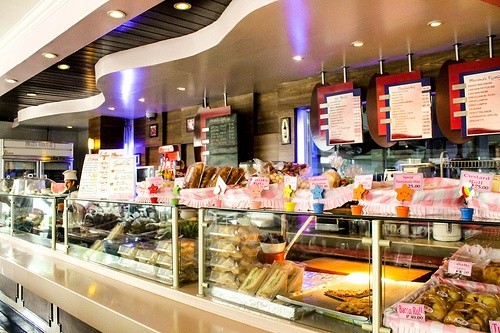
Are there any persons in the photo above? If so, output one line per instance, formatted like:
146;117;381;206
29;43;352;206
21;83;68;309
57;170;78;228
434;151;460;179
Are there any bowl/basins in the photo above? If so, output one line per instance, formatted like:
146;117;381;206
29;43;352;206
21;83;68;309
433;222;461;242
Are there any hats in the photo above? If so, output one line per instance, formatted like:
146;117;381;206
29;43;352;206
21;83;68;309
62;170;77;181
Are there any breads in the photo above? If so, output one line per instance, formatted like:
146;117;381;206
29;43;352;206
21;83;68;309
118;242;195;265
184;164;339;189
88;223;123;251
413;265;500;332
216;226;259;284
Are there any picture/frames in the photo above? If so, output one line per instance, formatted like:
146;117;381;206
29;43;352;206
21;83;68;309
186;117;194;132
280;117;291;145
149;124;158;137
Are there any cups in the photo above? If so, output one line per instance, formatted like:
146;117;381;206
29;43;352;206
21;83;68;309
257;251;285;264
260;241;286;254
385;225;432;238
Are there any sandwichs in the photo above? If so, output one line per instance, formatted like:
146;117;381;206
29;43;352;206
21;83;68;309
241;261;303;295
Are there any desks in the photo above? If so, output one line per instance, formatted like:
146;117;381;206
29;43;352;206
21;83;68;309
303;256;432;282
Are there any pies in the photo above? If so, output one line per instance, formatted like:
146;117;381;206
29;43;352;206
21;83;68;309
325;289;372;316
